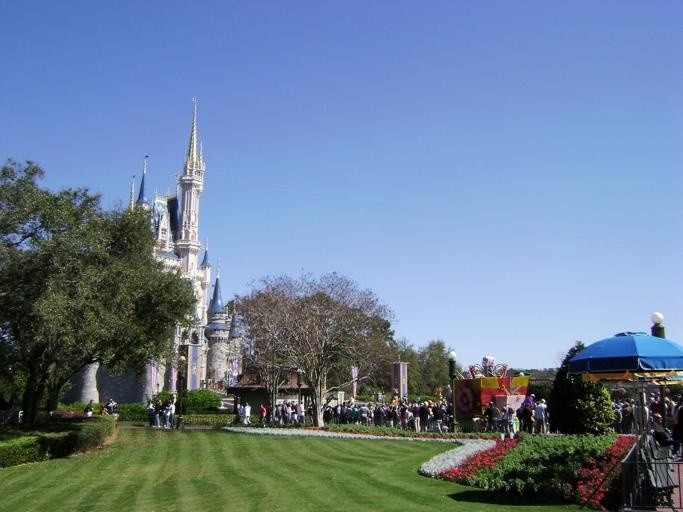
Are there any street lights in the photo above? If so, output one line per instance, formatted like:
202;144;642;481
175;355;186;429
225;368;232;387
447;351;457;434
649;311;667;429
208;366;212;379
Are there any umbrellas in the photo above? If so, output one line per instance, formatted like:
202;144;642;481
567;333;683;391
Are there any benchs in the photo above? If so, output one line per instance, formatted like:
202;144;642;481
637;424;679;512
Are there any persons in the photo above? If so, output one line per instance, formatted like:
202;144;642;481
517;394;547;434
233;397;251;424
349;395;355;407
611;391;683;455
325;400;449;434
483;401;514;433
259;404;272;423
146;394;182;429
84;400;94;416
100;399;117;415
